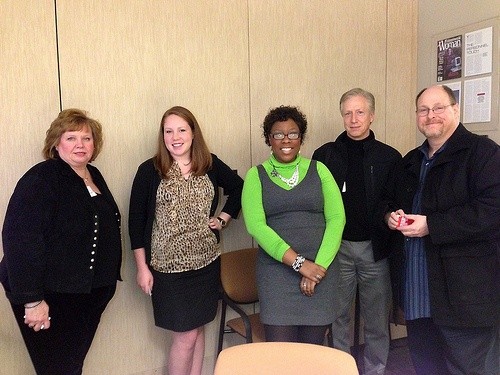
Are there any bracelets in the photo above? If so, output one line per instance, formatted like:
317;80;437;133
290;253;306;273
23;300;43;309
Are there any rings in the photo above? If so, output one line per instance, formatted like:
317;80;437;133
315;274;320;279
303;282;307;286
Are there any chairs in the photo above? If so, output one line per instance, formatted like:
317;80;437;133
214;339;361;375
215;248;266;358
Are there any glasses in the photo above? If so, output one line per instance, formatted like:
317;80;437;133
270;132;302;140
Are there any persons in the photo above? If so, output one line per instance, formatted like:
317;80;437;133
0;108;125;375
126;105;246;374
241;105;347;346
363;84;500;375
309;89;405;375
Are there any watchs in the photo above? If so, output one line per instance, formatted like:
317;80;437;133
217;217;226;230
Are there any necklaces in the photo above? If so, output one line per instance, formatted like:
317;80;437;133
267;155;301;187
70;166;88;181
